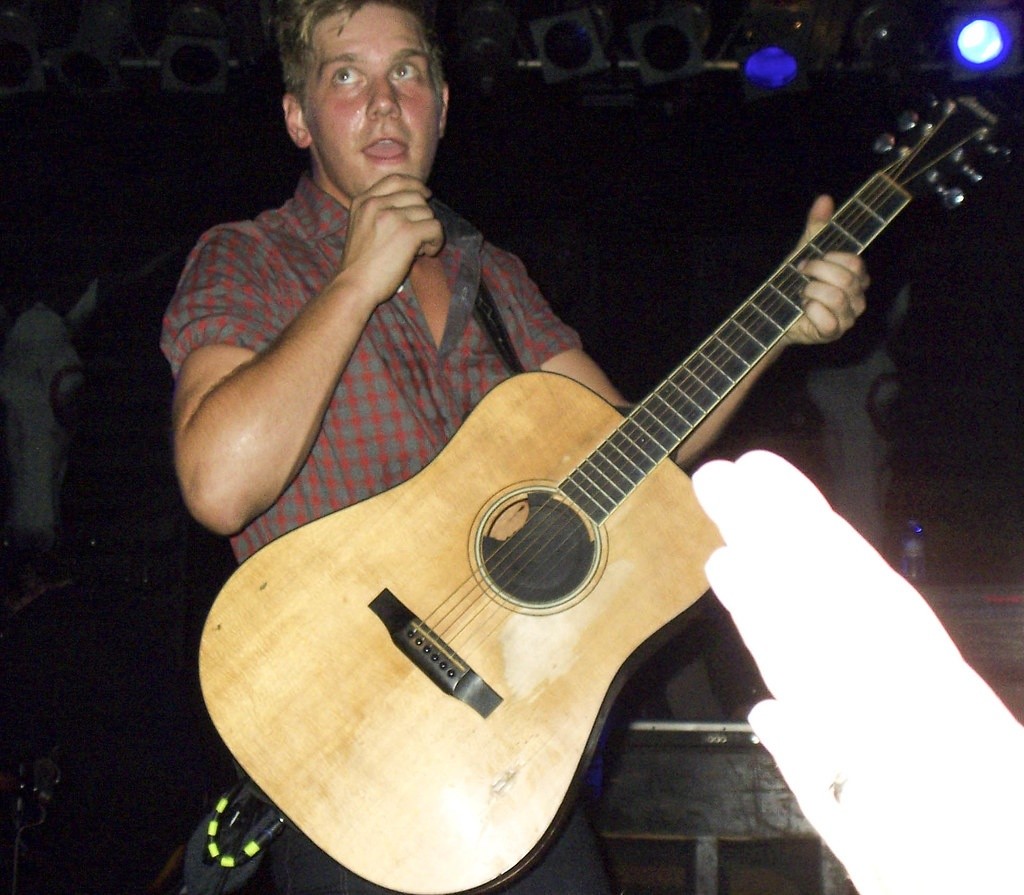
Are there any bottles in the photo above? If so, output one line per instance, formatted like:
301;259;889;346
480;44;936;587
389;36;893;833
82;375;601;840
900;538;937;613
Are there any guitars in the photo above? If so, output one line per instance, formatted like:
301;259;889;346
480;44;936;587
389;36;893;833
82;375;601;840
194;92;1016;893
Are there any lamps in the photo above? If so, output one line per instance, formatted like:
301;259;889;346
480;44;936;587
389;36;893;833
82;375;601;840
528;0;611;84
851;0;924;73
629;0;709;85
53;0;134;95
0;0;42;96
947;0;1024;79
161;0;229;94
733;0;844;102
459;0;518;70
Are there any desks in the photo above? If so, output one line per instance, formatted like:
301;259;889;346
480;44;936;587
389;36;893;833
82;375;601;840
592;586;1024;895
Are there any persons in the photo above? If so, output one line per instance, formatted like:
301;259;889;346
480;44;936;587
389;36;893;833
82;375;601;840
162;0;870;895
0;275;87;570
